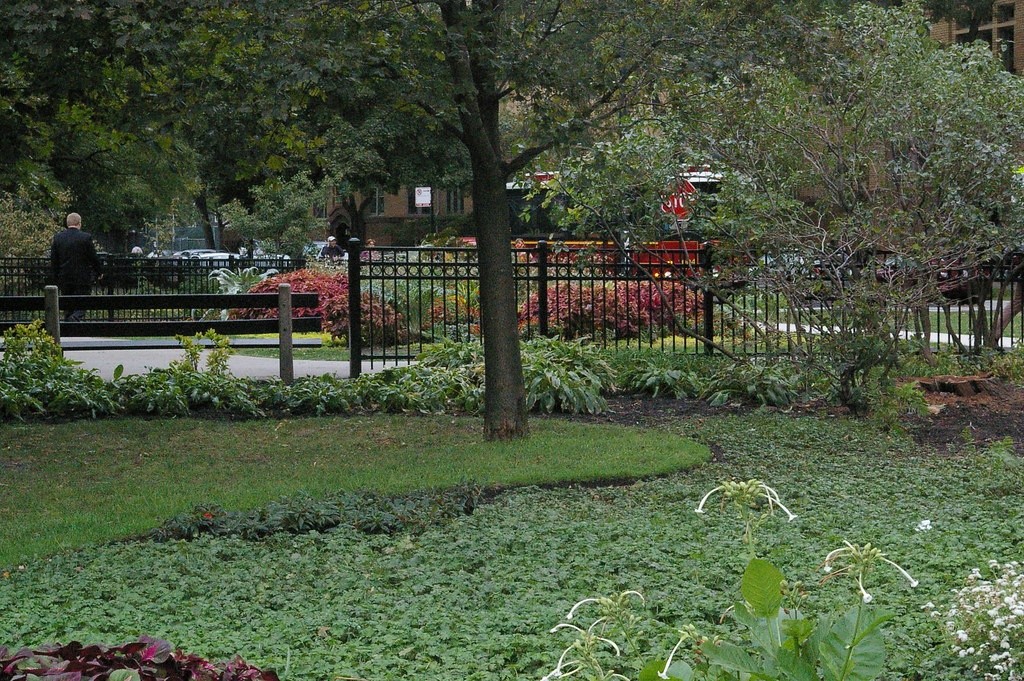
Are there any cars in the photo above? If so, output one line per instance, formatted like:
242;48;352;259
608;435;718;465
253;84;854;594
133;242;348;272
747;252;993;301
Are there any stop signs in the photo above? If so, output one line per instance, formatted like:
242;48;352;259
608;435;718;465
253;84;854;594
659;177;701;222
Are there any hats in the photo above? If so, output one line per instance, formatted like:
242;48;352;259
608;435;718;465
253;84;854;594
328;235;336;242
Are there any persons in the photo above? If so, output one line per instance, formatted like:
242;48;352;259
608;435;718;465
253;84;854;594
322;236;345;258
50;211;104;323
360;238;382;261
511;238;533;263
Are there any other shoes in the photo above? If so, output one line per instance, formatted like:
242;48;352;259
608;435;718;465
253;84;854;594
67;316;78;322
